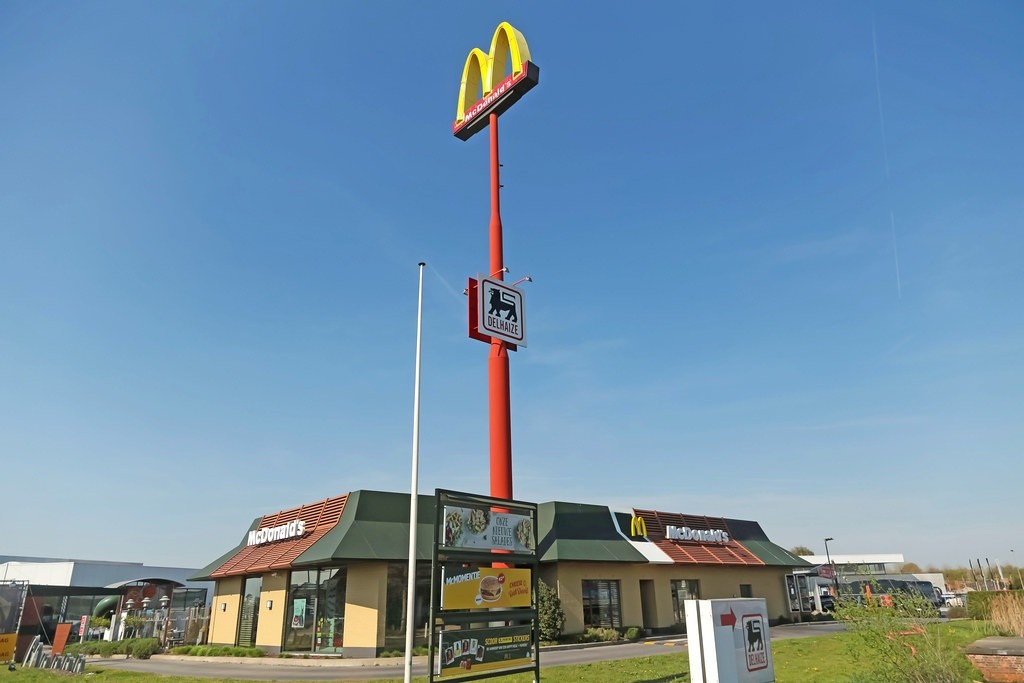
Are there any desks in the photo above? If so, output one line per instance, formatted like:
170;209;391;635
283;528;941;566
171;631;184;638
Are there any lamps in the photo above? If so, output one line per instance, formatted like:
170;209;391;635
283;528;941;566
159;595;170;606
125;599;135;609
221;603;226;612
141;597;151;609
193;598;200;607
267;600;272;609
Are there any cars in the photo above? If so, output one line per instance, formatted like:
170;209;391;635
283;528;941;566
945;596;964;608
808;594;840;612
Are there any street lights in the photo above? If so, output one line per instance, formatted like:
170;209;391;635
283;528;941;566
824;537;836;586
403;262;426;683
1010;548;1024;590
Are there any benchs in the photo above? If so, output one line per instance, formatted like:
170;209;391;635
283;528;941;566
168;639;184;647
